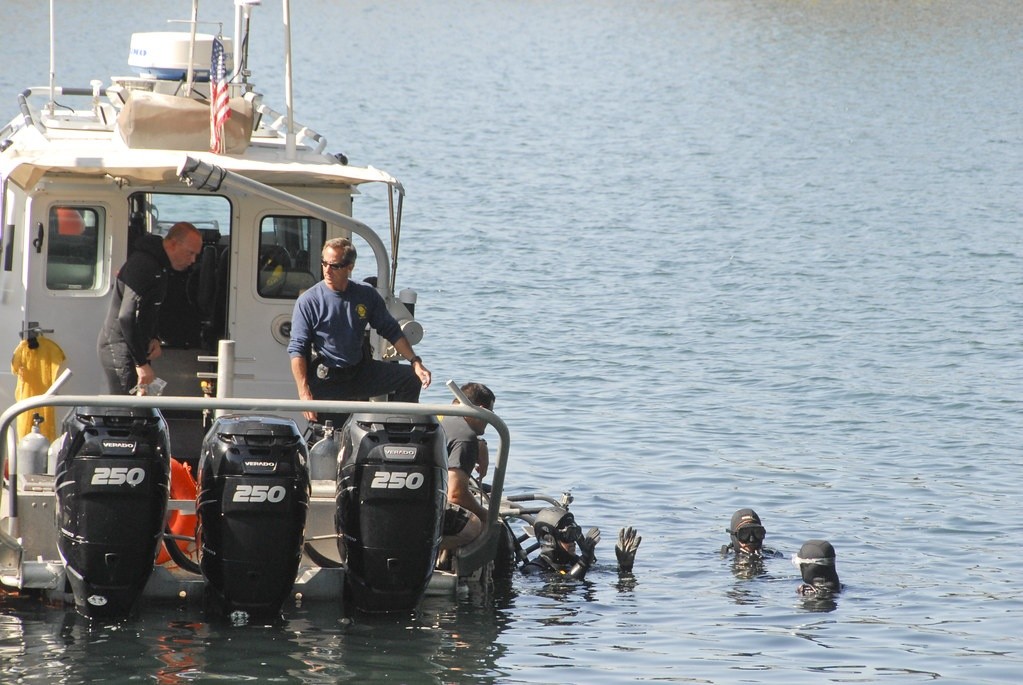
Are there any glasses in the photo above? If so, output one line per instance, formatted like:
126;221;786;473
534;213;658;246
558;523;582;541
321;259;350;270
792;553;811;568
735;524;766;542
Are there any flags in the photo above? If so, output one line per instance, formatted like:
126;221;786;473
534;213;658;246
210;35;232;154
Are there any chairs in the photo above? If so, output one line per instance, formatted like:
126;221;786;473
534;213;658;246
129;225;311;351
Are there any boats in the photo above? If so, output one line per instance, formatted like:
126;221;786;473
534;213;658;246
1;0;573;623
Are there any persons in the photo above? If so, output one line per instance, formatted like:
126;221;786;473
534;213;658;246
720;508;783;557
791;539;845;594
96;221;203;395
286;237;432;451
520;506;642;578
441;382;514;576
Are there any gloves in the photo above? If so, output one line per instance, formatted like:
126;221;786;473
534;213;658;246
582;528;601;566
615;526;641;571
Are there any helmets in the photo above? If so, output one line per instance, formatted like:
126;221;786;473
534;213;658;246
56;208;87;236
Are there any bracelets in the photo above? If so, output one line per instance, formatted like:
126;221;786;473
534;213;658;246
410;356;422;364
